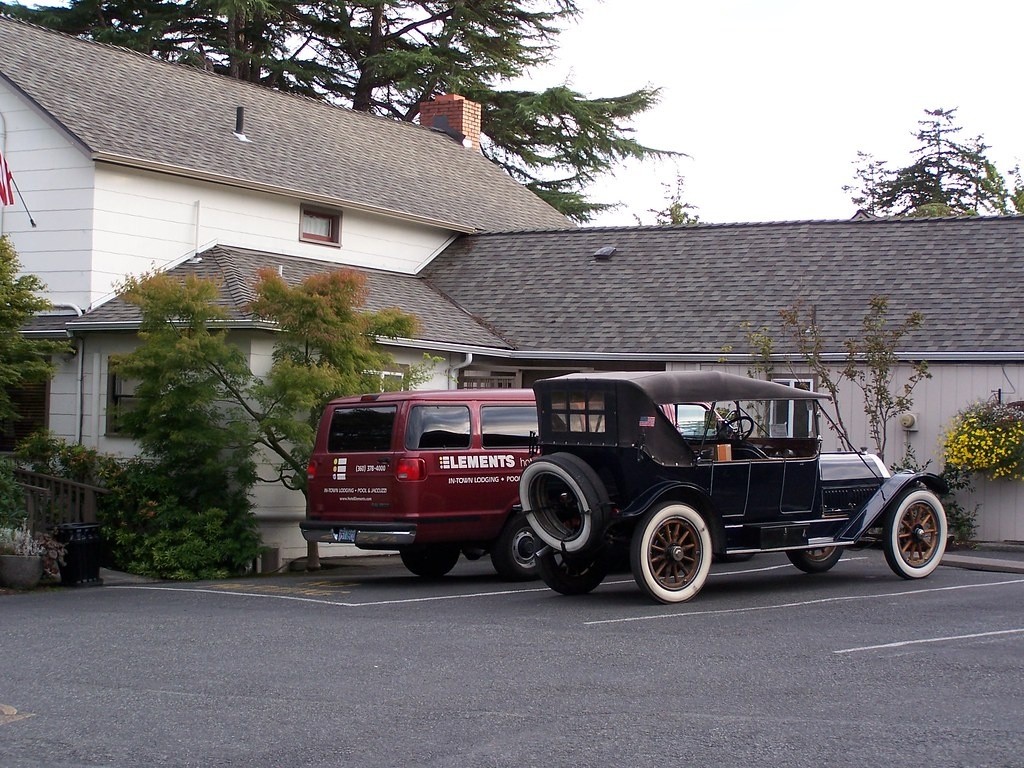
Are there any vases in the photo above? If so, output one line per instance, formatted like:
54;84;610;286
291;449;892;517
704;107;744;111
0;554;42;590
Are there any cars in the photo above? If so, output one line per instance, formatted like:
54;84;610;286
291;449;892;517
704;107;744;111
514;371;949;605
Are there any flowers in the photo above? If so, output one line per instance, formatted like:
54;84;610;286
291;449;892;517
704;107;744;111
0;517;46;555
934;397;1024;483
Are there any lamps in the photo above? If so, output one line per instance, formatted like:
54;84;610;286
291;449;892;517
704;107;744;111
901;411;920;432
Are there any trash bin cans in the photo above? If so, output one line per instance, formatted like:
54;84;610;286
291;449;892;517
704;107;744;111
47;522;104;587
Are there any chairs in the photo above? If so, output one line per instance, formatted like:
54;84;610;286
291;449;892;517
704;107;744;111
727;439;767;458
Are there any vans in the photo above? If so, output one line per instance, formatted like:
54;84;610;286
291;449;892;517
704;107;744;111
298;384;740;583
